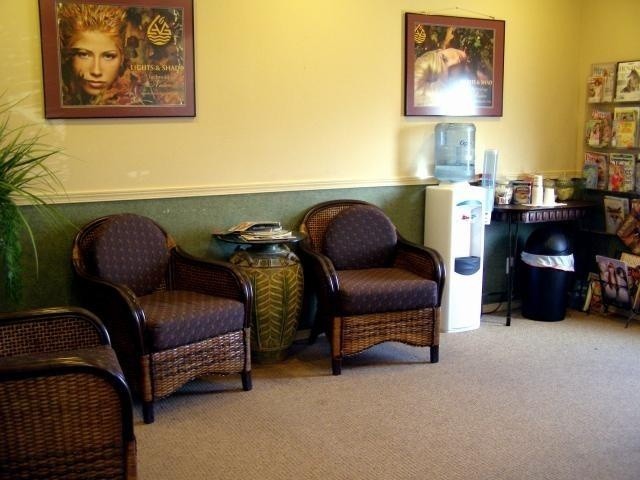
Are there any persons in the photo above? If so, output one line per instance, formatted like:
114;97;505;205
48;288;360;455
56;3;140;106
414;49;494;112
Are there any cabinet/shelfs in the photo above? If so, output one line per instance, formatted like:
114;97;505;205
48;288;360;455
583;58;640;329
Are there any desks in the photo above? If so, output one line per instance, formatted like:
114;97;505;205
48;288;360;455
210;223;311;369
479;192;591;324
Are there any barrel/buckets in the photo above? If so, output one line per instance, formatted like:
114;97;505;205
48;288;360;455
434;123;476;183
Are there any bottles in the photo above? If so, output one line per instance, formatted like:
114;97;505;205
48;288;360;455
568;178;586;201
495;180;514;205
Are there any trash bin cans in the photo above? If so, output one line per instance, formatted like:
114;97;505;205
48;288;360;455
521;228;571;322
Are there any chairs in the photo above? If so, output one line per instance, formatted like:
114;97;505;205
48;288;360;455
2;304;141;479
298;196;447;377
67;211;257;424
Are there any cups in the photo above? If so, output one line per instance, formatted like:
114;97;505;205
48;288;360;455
531;174;555;206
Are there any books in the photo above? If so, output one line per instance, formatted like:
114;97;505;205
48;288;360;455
581;61;639;317
214;220;298;240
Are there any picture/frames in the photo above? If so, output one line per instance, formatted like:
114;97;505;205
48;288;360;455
37;0;199;121
405;10;507;118
